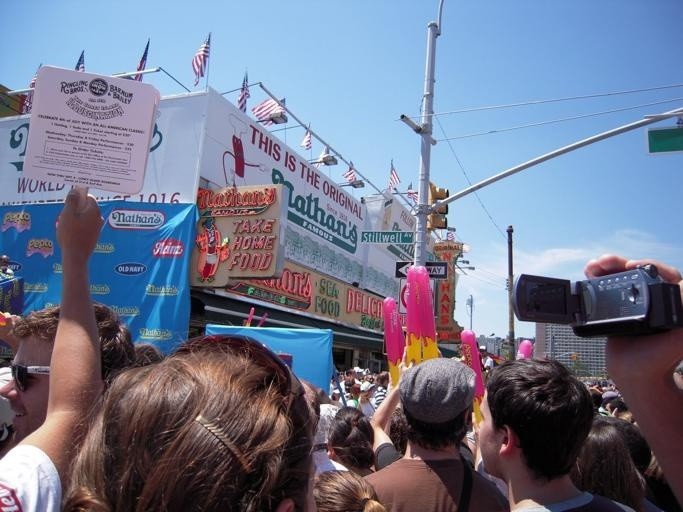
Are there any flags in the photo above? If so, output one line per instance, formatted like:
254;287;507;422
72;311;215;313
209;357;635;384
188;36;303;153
21;63;42;116
341;161;356;183
251;96;284;128
74;50;84;72
412;192;418;203
387;167;401;188
237;74;249;113
191;33;210;85
132;40;150;82
298;126;311;150
407;184;411;198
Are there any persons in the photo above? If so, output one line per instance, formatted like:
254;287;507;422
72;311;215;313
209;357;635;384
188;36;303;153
582;254;682;511
0;186;109;512
0;298;681;512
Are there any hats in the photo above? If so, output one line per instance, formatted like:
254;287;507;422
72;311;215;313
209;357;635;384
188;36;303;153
354;366;375;392
601;391;619;400
399;358;475;424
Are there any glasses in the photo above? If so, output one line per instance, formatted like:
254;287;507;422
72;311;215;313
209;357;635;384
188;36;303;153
10;361;51;391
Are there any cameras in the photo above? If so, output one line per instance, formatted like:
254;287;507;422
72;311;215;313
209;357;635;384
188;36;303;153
510;263;682;338
